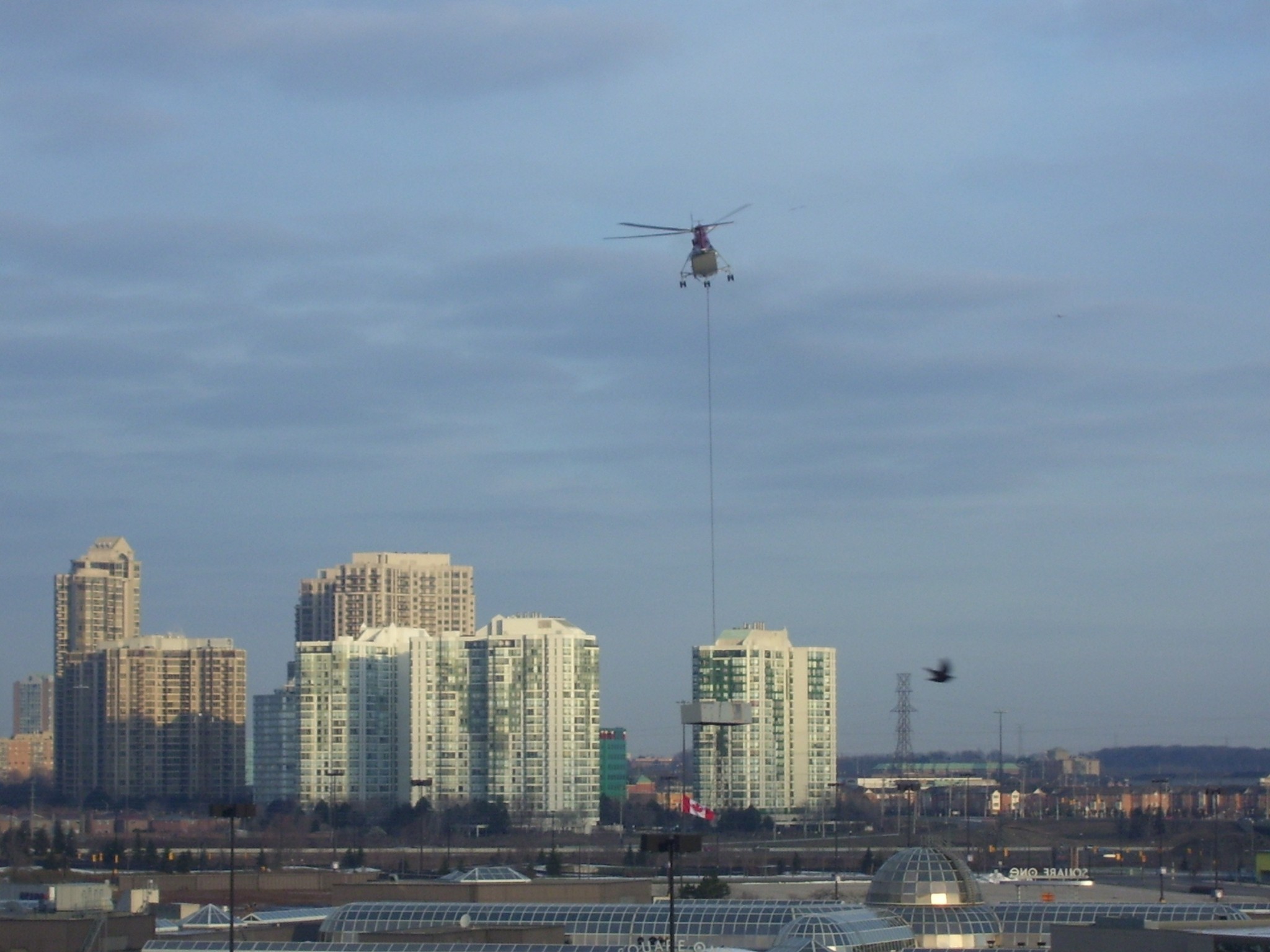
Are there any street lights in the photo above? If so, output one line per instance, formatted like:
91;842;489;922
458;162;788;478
1150;778;1170;904
219;804;240;952
989;709;1008;839
826;782;847;900
321;768;346;862
959;771;978;860
895;781;922;848
1205;788;1222;895
406;776;432;878
637;828;704;952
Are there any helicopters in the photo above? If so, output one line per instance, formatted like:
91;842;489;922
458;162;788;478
603;193;757;287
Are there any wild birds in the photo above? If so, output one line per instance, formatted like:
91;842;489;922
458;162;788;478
923;658;956;682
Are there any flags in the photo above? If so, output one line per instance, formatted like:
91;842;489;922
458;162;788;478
681;794;715;821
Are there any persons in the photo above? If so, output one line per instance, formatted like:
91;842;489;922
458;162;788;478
637;935;669;946
